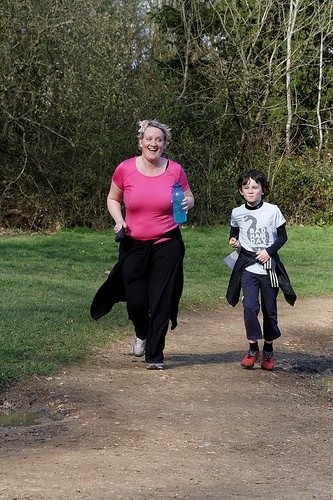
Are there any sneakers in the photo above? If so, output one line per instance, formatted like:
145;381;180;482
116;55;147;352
146;362;164;370
262;352;275;370
133;337;146;357
240;349;261;368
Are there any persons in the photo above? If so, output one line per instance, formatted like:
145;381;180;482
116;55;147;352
89;121;195;370
225;169;298;371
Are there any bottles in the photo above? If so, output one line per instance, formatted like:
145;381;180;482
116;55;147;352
172;182;188;223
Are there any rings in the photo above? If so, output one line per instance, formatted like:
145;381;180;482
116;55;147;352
115;227;117;228
